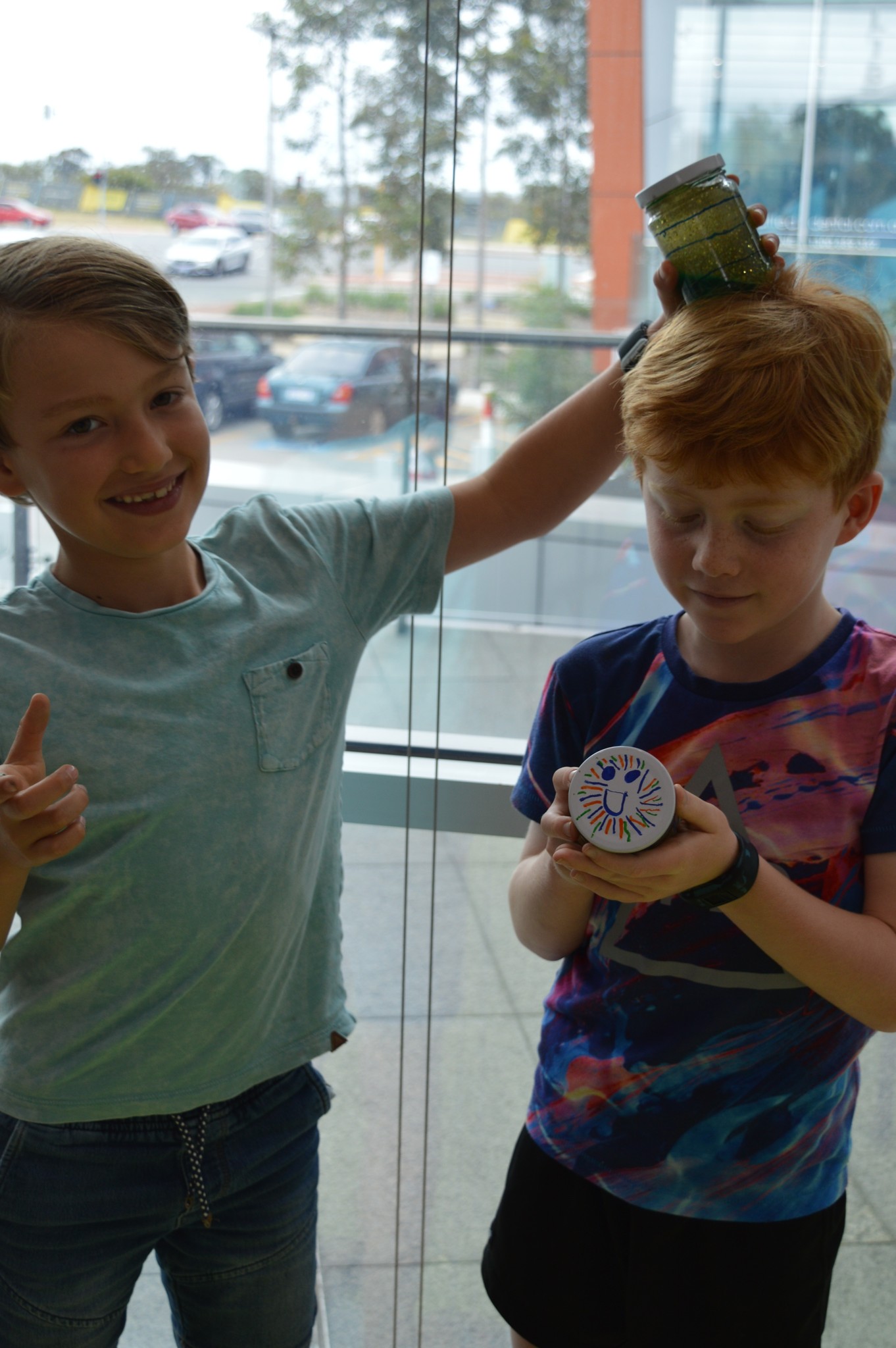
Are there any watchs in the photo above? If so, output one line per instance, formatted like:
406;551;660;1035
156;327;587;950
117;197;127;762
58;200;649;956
694;828;759;915
617;319;656;375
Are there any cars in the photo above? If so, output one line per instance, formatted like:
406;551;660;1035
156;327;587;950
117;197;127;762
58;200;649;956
0;194;54;226
249;336;460;443
164;202;316;279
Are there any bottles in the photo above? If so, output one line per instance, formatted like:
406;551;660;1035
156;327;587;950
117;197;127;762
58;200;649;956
636;152;773;309
569;745;680;858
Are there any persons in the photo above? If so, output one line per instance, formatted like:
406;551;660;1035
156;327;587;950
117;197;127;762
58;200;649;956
480;264;896;1348
0;174;790;1348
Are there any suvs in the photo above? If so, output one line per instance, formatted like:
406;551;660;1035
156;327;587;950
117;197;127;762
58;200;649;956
183;327;282;431
